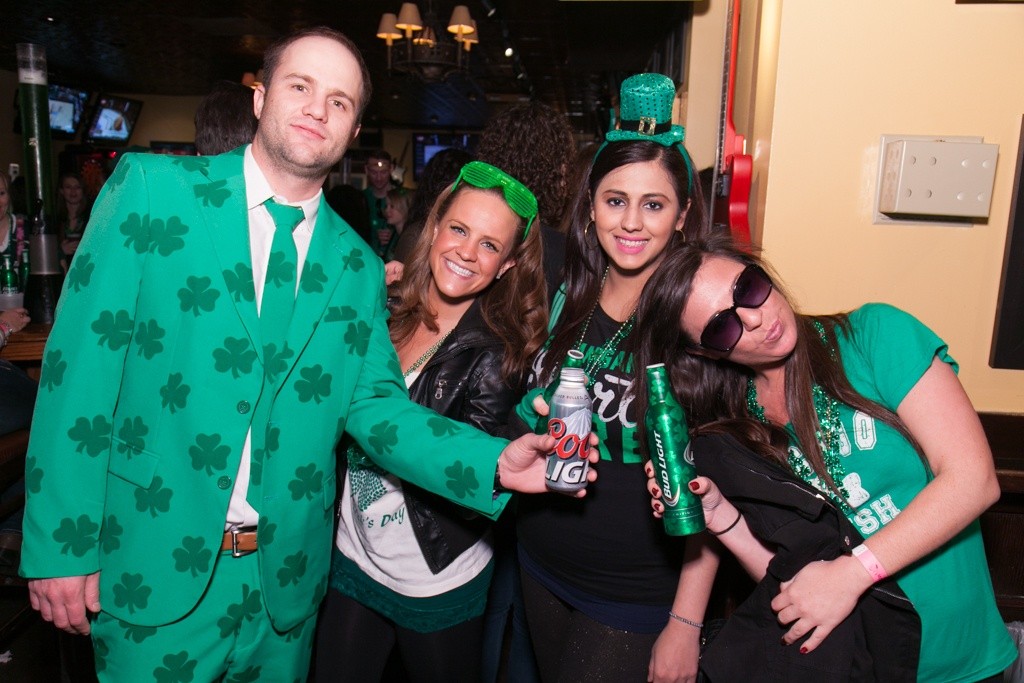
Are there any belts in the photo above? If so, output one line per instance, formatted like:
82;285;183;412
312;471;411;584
220;525;259;558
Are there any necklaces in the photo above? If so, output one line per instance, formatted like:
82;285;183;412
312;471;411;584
558;261;657;393
402;326;456;380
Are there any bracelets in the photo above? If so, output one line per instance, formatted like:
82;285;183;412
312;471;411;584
714;512;742;538
0;319;12;346
669;612;704;629
852;544;887;583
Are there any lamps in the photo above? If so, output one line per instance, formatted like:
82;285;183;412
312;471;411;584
479;1;539;96
377;2;479;83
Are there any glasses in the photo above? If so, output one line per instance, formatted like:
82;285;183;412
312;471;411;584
697;264;773;354
452;161;538;242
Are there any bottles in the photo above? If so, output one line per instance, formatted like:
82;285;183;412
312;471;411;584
19;248;29;292
644;362;706;537
1;253;18;295
536;349;584;436
380;222;388;254
544;368;592;492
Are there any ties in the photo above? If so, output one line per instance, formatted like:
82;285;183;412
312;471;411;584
246;198;306;515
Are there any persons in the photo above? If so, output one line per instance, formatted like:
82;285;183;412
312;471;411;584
55;170;92;276
638;239;1019;683
363;150;409;258
512;128;721;683
385;186;419;262
475;102;583;316
17;26;600;683
194;89;258;158
0;171;34;293
327;184;380;254
0;308;31;352
321;161;550;682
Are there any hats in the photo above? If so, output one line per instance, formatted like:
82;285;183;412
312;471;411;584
605;73;684;147
